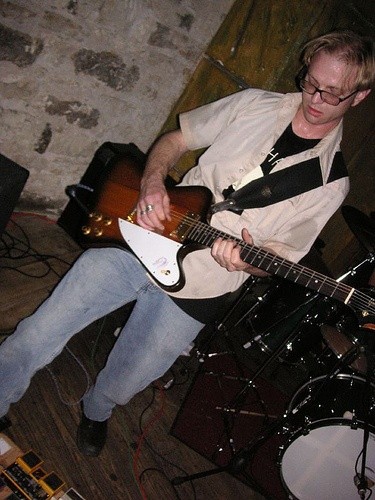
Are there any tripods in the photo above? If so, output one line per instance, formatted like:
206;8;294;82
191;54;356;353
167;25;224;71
171;250;375;500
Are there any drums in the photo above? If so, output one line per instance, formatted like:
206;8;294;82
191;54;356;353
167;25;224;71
277;374;374;500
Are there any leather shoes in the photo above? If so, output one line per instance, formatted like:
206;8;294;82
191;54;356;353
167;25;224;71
78;399;108;459
0;414;12;432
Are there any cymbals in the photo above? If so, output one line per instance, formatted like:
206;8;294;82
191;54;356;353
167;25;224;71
319;323;371;373
341;204;374;257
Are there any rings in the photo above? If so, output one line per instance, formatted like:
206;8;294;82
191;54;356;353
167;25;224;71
146;205;154;212
141;211;146;216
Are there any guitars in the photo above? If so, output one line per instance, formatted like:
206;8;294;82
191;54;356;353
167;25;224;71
56;140;375;318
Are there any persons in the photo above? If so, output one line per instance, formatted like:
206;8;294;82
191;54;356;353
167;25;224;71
0;32;371;458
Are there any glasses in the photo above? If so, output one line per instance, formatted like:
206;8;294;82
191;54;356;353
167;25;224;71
297;64;359;106
